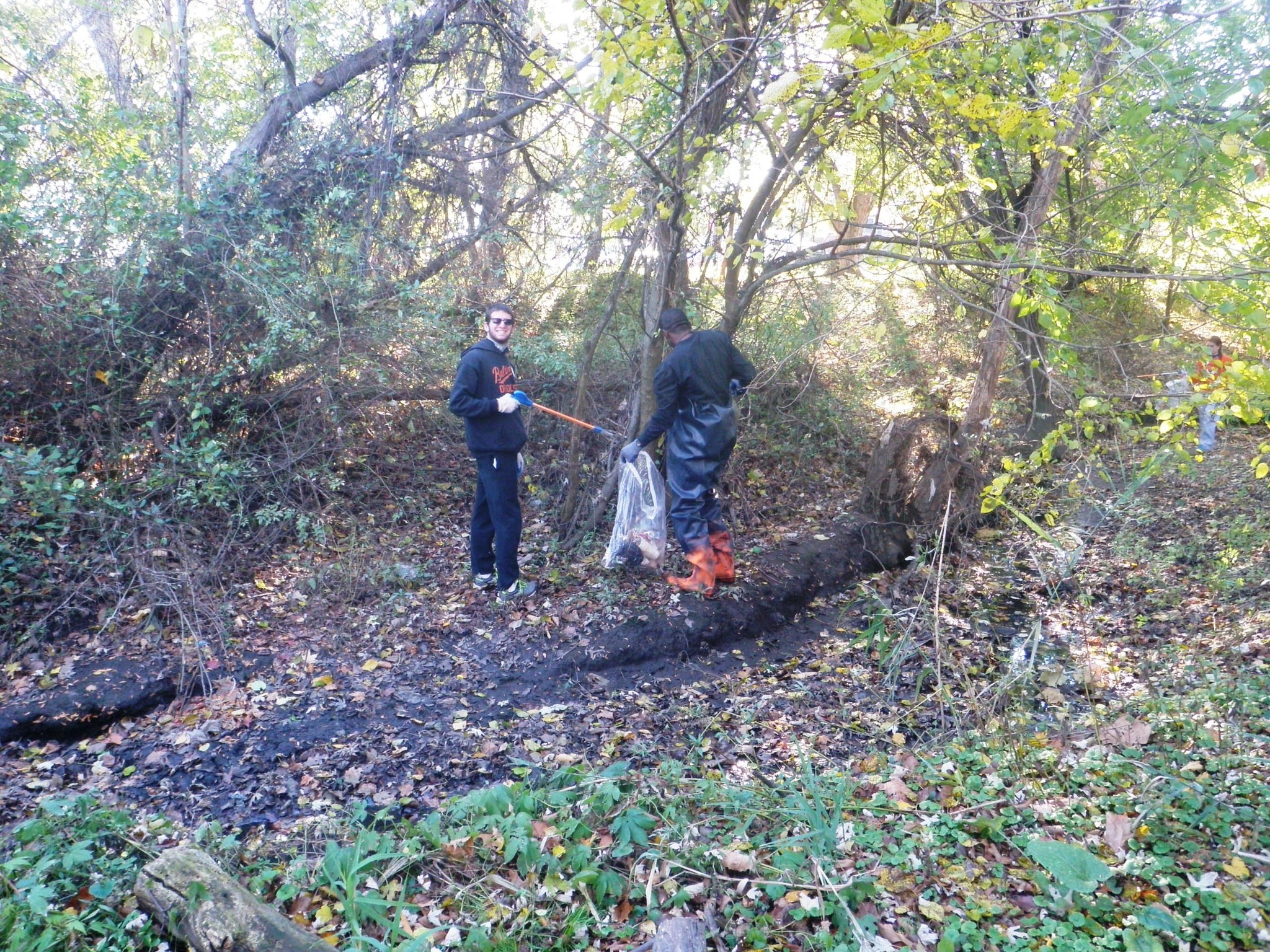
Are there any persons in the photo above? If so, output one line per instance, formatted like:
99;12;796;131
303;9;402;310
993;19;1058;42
1182;332;1234;453
617;303;757;603
447;300;543;603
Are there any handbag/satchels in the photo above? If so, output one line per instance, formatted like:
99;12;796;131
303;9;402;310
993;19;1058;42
601;450;667;576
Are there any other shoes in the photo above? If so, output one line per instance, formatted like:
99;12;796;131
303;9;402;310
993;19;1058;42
1198;444;1209;452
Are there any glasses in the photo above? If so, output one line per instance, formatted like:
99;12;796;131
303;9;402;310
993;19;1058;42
488;317;515;325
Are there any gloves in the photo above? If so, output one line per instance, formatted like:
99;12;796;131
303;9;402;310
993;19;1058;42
496;393;521;414
516;452;524;477
620;438;643;464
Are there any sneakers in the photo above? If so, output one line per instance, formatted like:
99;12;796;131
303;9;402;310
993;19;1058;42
472;568;498;590
495;578;538;606
